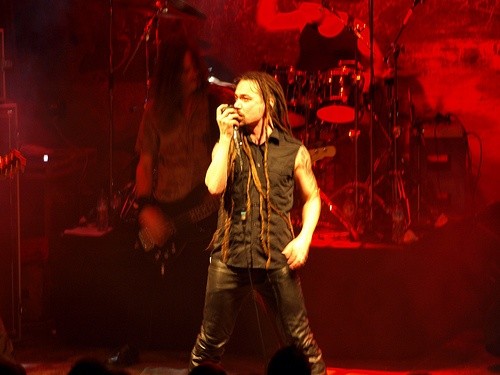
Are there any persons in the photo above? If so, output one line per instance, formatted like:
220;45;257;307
256;0;385;94
191;72;327;375
108;33;233;366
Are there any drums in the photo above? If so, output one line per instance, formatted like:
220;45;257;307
316;68;359;122
267;68;310;127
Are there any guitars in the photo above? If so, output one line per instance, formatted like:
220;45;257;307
134;195;222;253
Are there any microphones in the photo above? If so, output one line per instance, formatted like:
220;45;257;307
221;107;238;138
413;0;420;8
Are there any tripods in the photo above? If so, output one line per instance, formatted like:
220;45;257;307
349;0;424;243
